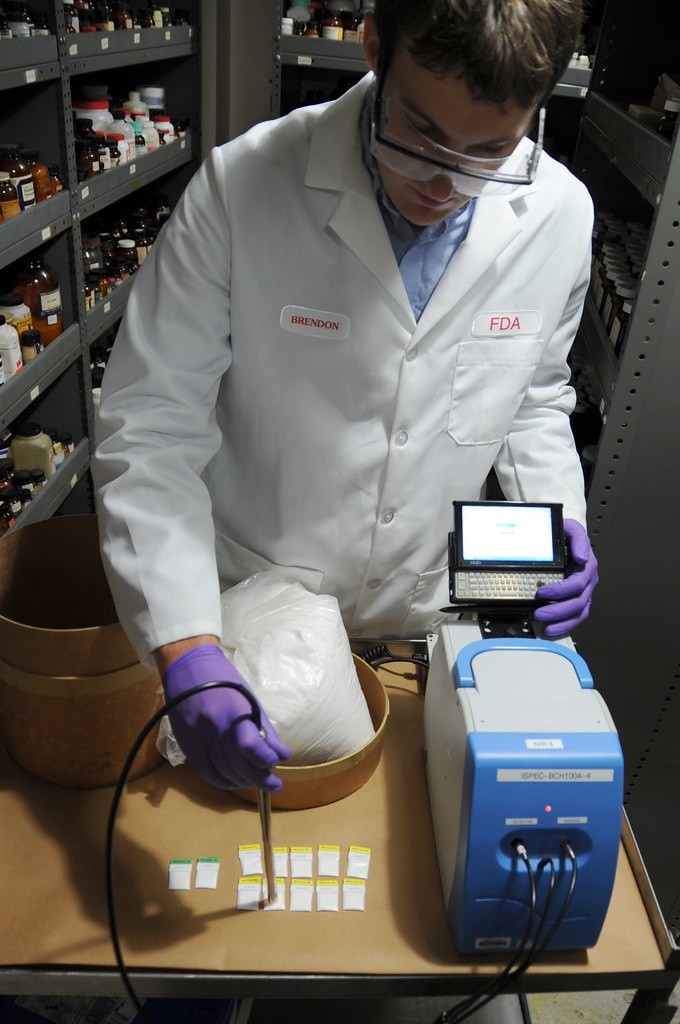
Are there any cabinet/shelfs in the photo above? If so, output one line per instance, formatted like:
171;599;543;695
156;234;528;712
0;0;232;539
570;0;680;946
269;0;593;124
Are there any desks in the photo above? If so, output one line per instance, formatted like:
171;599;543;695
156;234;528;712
0;637;680;1024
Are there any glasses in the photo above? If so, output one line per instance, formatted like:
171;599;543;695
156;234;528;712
369;70;545;196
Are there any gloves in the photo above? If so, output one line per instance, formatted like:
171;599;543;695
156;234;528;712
535;518;599;634
162;645;290;793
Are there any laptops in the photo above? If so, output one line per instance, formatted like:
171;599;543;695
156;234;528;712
451;500;566;604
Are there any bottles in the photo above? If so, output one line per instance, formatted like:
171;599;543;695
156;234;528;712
280;0;375;44
74;83;187;185
0;142;64;226
90;338;114;405
656;96;680;141
61;0;175;35
0;0;51;38
568;346;598;412
0;258;63;390
0;422;77;538
579;444;597;463
575;206;655;353
83;197;174;312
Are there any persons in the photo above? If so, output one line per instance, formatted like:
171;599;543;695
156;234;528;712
89;0;602;792
